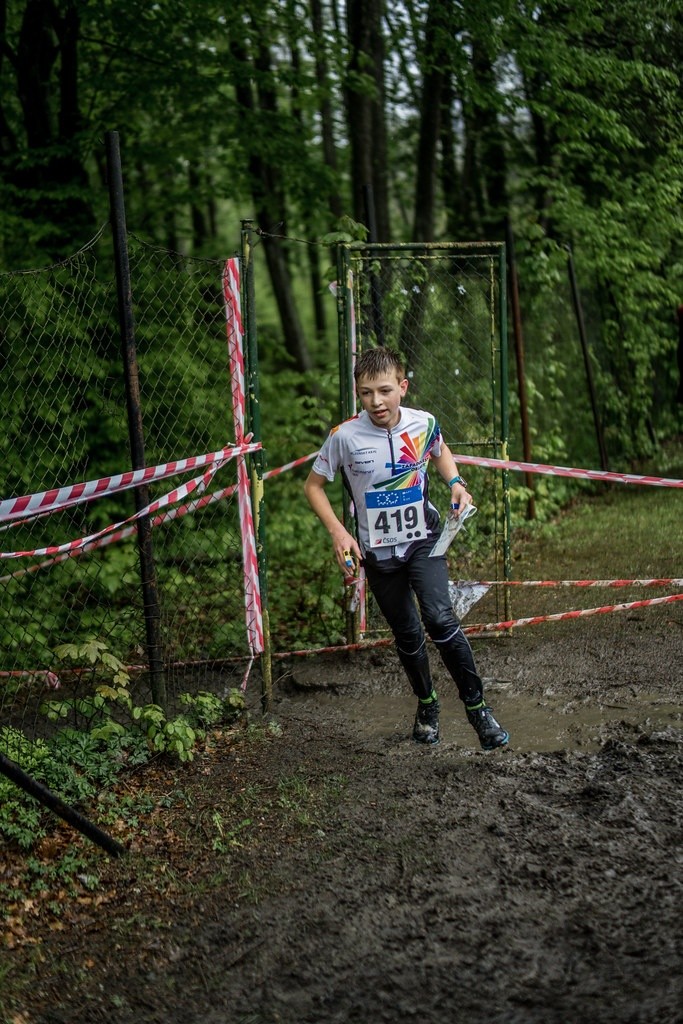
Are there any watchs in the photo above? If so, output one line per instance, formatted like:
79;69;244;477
448;475;467;489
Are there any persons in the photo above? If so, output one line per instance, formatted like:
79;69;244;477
303;348;513;751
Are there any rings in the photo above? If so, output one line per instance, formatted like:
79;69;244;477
342;549;354;567
449;503;459;510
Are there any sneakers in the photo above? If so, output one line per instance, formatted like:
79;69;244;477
465;698;510;750
412;689;440;744
